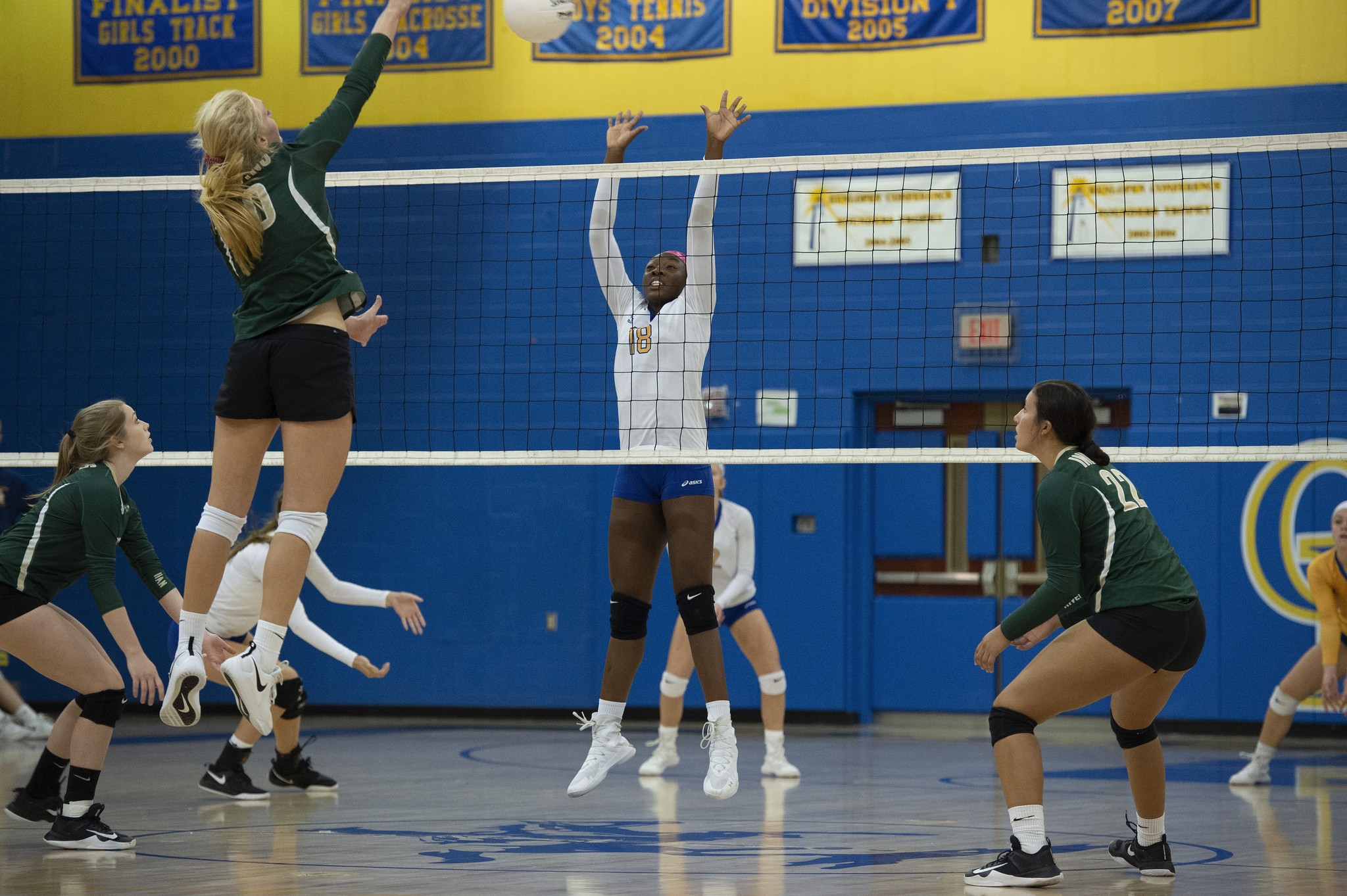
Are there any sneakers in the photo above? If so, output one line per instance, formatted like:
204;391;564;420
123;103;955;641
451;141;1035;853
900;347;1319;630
267;735;338;793
638;732;681;776
43;802;136;850
566;711;636;798
1108;810;1176;876
1228;751;1272;785
220;639;290;736
3;776;64;824
759;742;800;778
159;636;207;727
963;835;1063;887
700;712;739;800
197;763;271;801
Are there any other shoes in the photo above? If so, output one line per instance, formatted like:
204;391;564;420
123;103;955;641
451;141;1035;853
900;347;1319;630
1;710;55;741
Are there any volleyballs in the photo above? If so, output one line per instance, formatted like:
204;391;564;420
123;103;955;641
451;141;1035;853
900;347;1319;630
503;0;576;44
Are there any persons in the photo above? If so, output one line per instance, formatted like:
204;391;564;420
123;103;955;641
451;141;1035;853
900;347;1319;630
961;381;1210;886
158;0;424;803
0;397;236;851
1227;501;1347;788
555;88;802;797
